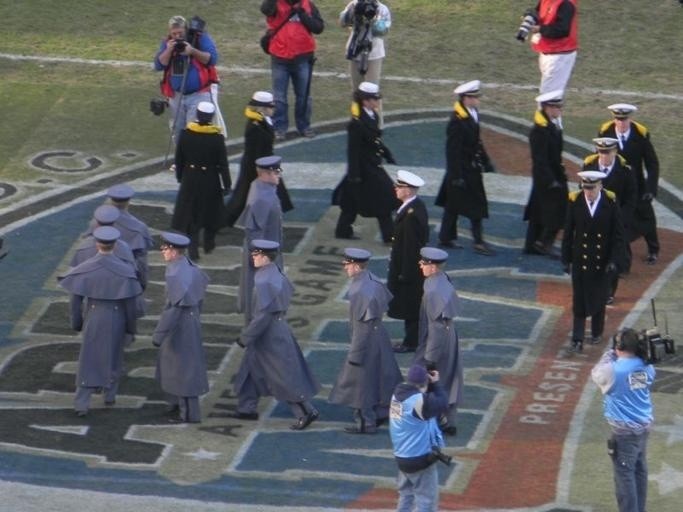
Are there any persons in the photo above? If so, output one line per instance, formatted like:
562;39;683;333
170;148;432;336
520;89;661;356
380;169;428;353
151;232;211;423
519;0;579;132
232;155;285;327
388;364;449;512
260;0;324;142
327;248;405;434
57;184;154;419
339;0;393;134
416;247;463;435
232;239;319;430
433;79;498;256
151;16;292;263
590;327;657;511
331;81;403;243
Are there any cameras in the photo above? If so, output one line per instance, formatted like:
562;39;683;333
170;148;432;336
173;37;186;52
362;1;375;18
515;11;538;41
425;363;436;374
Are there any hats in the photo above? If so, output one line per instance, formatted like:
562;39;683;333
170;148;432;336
408;365;428;382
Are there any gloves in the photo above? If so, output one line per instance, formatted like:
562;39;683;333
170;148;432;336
641;192;653;202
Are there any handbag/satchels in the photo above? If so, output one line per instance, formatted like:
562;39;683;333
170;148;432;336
261;29;274;54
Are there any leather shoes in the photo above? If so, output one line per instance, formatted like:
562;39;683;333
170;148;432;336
569;341;583;352
394;345;413;353
645;251;659;264
606;297;615;305
590;335;602;344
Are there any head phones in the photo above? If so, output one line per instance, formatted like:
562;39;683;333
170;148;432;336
616;329;625;352
